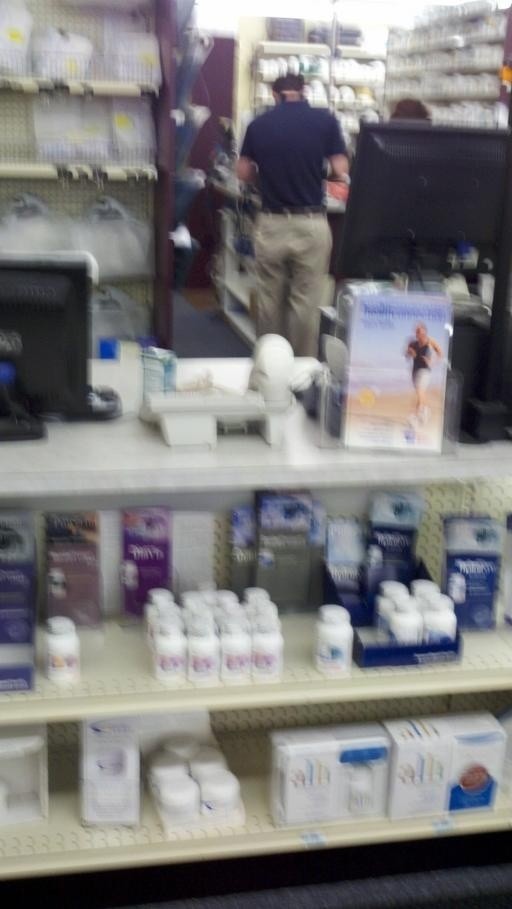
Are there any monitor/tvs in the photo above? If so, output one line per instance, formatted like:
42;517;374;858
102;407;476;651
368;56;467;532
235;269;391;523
0;255;94;440
333;117;511;287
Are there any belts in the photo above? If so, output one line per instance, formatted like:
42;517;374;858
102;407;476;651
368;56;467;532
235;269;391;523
262;204;324;215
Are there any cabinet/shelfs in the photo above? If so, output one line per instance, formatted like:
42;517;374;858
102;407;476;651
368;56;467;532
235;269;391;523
1;1;512;909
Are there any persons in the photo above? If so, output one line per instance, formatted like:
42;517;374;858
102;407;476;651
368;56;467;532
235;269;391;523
237;70;352;365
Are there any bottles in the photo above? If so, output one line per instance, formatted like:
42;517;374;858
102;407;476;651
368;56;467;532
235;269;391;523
43;614;80;683
144;587;284;686
372;574;460;645
314;603;355;674
